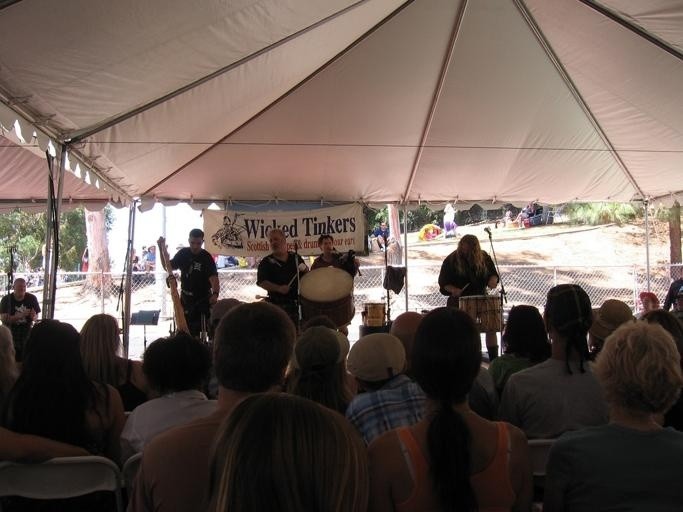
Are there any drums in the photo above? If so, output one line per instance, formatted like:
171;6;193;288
361;302;386;326
299;267;355;329
459;295;504;333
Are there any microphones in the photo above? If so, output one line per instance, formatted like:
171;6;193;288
383;234;388;247
484;227;491;235
294;240;298;255
128;240;131;250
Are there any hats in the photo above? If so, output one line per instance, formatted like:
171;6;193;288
544;284;595;343
211;297;247;327
346;332;407;383
587;298;638;341
392;312;423;343
639;292;661;304
294;325;351;371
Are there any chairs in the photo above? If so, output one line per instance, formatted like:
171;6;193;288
0;309;683;512
